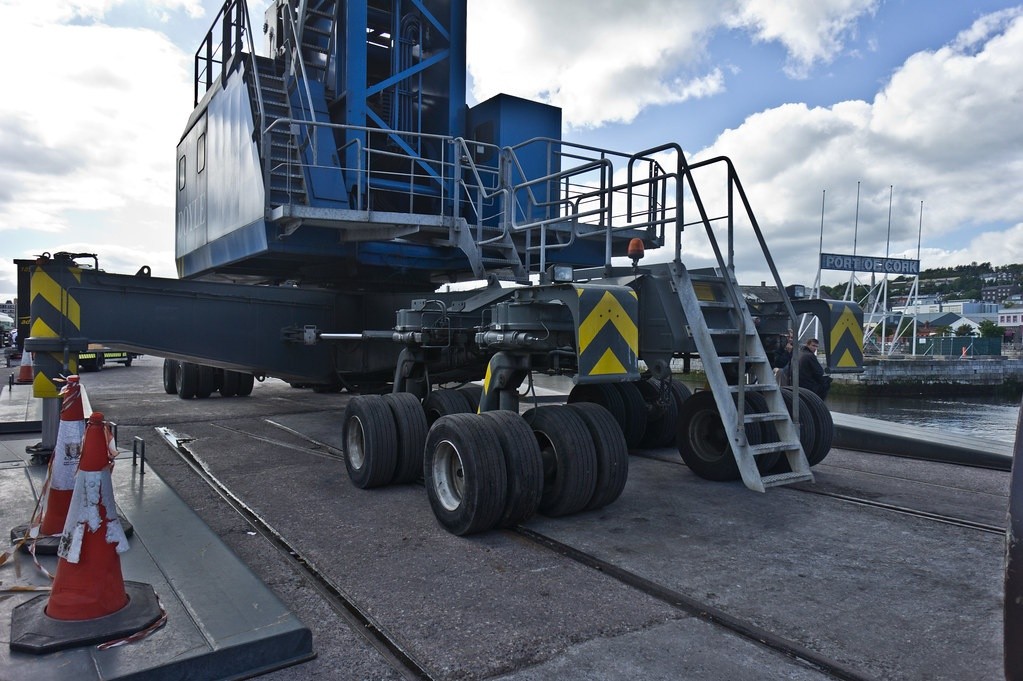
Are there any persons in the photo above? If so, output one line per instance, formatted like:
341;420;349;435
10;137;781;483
772;329;795;370
790;338;832;402
2;330;12;348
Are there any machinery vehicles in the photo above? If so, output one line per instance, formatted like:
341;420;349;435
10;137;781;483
24;1;865;537
10;374;133;556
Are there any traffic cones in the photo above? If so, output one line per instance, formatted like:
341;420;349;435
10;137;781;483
10;349;33;386
9;412;167;657
